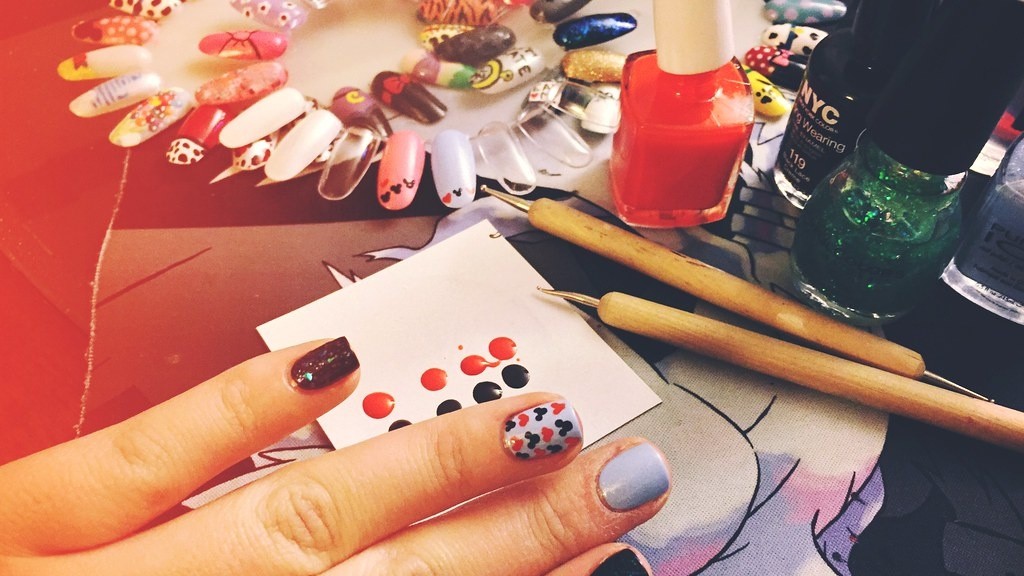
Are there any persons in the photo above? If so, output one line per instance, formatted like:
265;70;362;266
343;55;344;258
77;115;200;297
0;335;673;576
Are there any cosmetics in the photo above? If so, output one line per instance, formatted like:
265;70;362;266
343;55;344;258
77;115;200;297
608;0;756;230
773;0;1024;327
